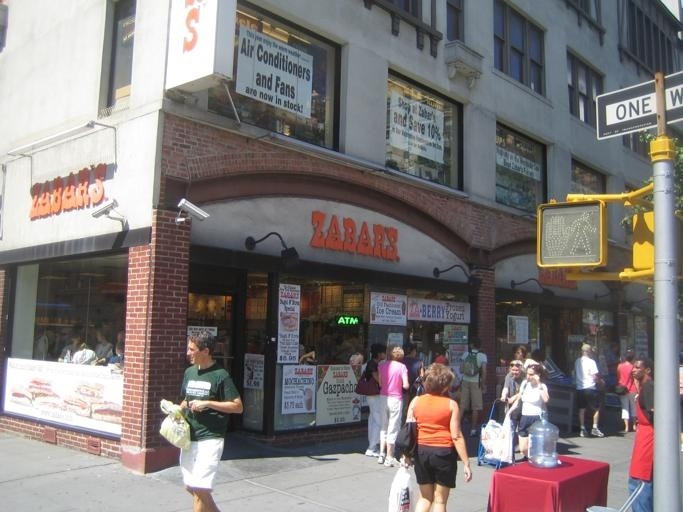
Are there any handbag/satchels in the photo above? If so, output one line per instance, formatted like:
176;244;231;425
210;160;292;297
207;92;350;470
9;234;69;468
614;385;628;395
511;399;523;421
395;421;415;459
354;372;381;396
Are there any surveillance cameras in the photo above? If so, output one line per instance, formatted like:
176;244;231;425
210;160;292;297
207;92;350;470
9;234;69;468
178;198;210;221
92;198;118;219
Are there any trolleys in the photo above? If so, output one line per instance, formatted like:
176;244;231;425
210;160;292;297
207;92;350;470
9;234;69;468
476;398;517;471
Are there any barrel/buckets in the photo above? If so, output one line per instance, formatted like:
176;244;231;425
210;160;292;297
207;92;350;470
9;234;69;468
526;410;560;468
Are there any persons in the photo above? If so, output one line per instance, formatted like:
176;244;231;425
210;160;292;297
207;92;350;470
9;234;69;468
177;330;244;512
34;318;124;365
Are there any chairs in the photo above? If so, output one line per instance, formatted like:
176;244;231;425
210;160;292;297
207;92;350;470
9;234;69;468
586;480;646;512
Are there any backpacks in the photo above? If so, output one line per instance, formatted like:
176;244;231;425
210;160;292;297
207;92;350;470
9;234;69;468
463;348;485;376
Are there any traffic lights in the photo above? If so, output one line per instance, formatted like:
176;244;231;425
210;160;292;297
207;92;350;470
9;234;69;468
532;194;613;293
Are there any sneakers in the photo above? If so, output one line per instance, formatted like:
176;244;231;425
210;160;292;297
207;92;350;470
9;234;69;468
591;429;605;438
580;429;590;437
365;448;399;468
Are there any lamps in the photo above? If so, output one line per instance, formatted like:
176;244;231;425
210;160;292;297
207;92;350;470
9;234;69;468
84;119;116;130
433;261;483;293
244;231;302;274
509;275;556;301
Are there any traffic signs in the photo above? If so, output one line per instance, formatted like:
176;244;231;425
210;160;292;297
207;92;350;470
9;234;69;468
593;66;682;143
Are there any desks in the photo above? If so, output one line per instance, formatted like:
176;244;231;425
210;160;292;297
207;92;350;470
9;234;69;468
486;455;611;512
